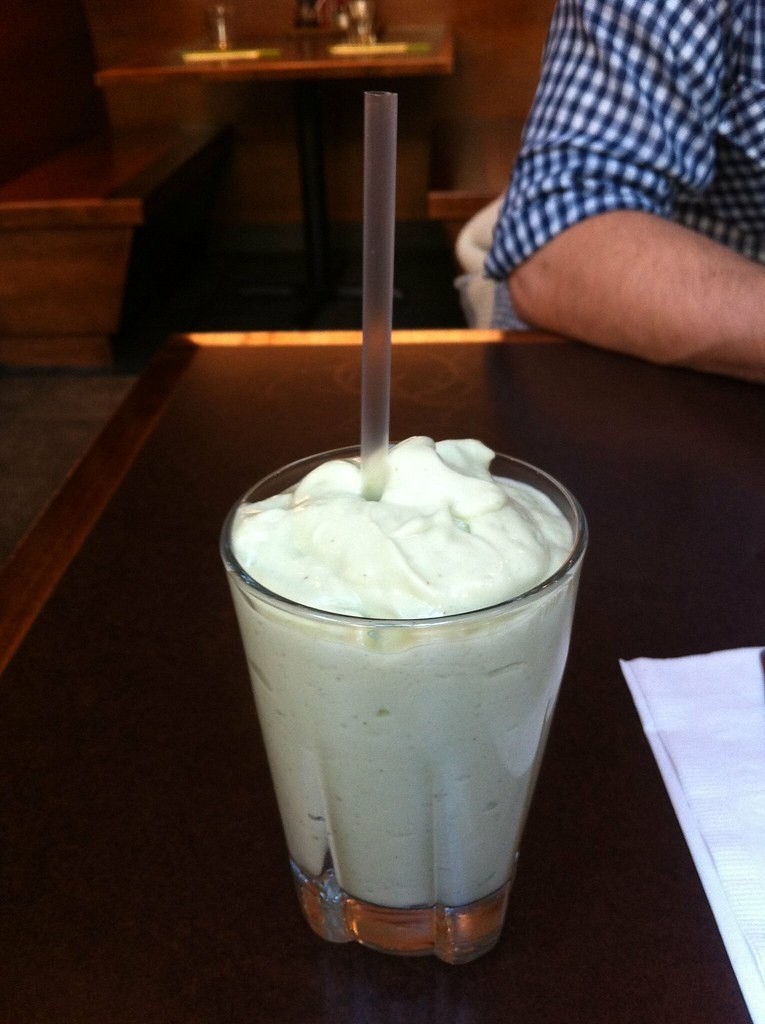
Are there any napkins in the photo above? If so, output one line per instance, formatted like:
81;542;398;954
618;648;765;1024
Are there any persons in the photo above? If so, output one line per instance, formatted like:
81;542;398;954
485;0;765;383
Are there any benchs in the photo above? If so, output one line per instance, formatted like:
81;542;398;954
429;117;536;239
0;0;227;371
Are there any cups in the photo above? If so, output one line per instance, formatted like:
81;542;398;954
343;1;379;47
221;432;588;965
205;2;237;52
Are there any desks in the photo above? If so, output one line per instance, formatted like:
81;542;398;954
0;334;765;1024
94;29;453;331
